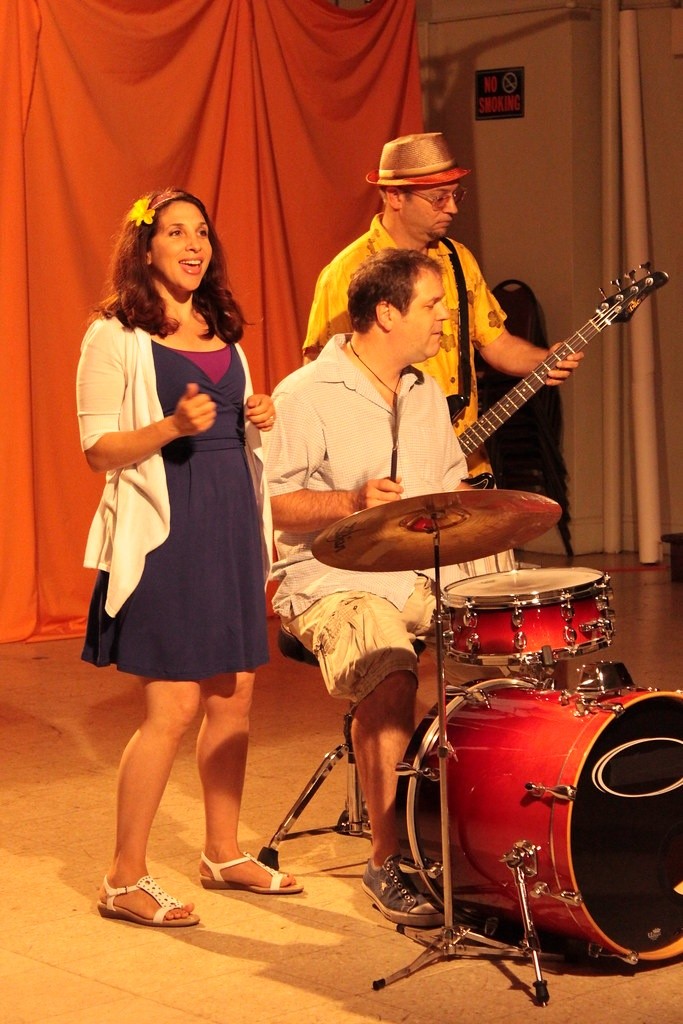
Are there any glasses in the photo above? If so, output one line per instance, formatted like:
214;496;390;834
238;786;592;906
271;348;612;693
400;186;466;211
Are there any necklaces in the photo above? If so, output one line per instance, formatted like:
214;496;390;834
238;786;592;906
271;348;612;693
349;340;396;395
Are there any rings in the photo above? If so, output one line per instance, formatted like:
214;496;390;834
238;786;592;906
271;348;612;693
269;417;275;424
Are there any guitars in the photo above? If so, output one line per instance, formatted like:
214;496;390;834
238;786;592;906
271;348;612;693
446;262;670;490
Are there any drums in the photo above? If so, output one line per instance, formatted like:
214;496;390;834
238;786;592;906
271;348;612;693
394;676;683;965
439;566;615;666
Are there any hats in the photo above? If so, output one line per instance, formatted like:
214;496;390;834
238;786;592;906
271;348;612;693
366;133;472;186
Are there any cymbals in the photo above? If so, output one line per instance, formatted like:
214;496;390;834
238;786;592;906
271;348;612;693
312;488;561;573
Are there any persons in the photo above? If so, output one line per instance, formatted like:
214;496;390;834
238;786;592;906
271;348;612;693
300;130;587;573
255;247;473;928
76;185;306;928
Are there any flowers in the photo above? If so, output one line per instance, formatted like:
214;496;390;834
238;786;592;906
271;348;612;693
127;196;155;227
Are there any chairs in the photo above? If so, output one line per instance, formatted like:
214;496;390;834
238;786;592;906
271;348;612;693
475;278;575;560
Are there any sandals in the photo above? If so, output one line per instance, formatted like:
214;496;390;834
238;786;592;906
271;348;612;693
200;852;303;894
97;874;200;927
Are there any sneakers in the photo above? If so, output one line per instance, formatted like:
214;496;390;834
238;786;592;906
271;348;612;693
361;853;447;926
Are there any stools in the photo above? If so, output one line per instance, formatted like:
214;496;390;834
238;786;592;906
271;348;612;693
269;620;427;853
